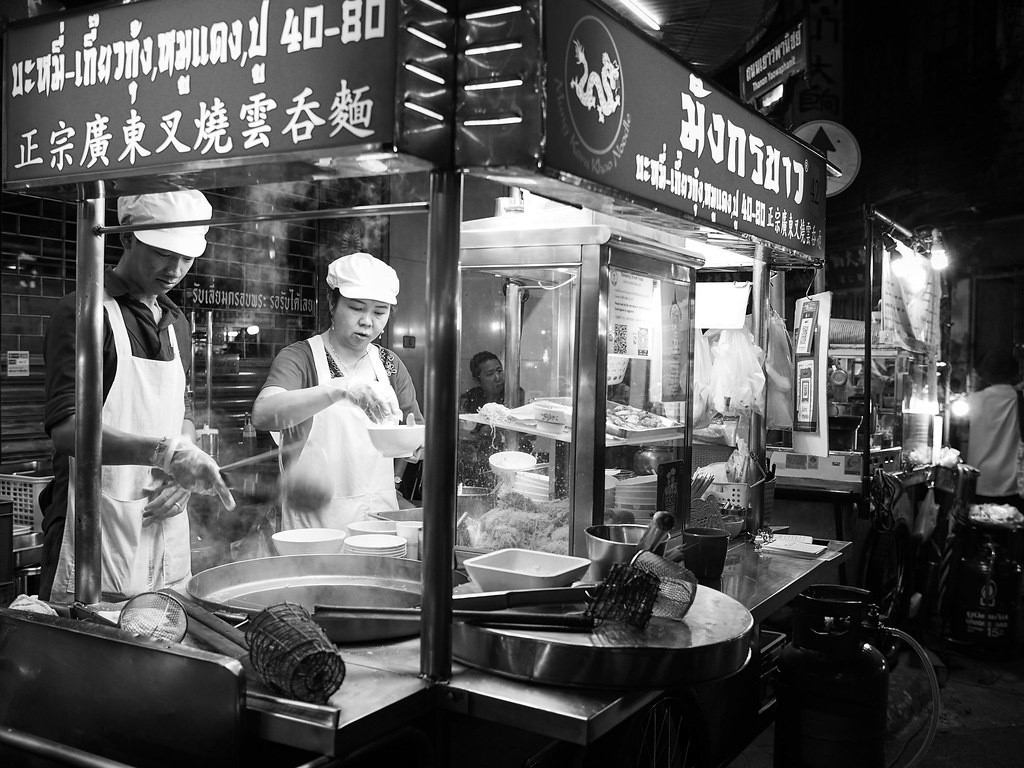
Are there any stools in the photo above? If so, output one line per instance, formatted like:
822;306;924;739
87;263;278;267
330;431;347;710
944;500;1024;684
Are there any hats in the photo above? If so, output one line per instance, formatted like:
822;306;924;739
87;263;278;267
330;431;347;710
326;253;400;305
117;188;212;257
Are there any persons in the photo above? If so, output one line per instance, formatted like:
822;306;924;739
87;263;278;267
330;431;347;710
458;350;538;487
250;252;427;533
38;189;235;608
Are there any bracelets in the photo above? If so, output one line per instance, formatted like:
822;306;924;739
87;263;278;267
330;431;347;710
153;436;167;466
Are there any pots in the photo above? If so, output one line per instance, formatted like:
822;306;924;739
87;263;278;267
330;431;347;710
459;486;499;520
186;554;472;645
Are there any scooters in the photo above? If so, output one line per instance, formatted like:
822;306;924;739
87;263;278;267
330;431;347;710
961;482;1024;658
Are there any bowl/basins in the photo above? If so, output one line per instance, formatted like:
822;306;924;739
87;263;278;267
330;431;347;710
722;520;744;540
489;450;658;524
463;547;592;593
535;403;567;434
584;524;672;580
367;425;425;458
271;520;424;560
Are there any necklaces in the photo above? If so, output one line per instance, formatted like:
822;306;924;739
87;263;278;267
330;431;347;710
328;328;367;374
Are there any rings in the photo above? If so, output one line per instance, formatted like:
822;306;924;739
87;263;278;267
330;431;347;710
175;502;182;509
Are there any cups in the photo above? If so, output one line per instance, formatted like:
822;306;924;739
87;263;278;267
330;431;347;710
682;528;731;581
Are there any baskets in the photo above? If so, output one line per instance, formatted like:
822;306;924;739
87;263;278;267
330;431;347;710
702;482;751;510
